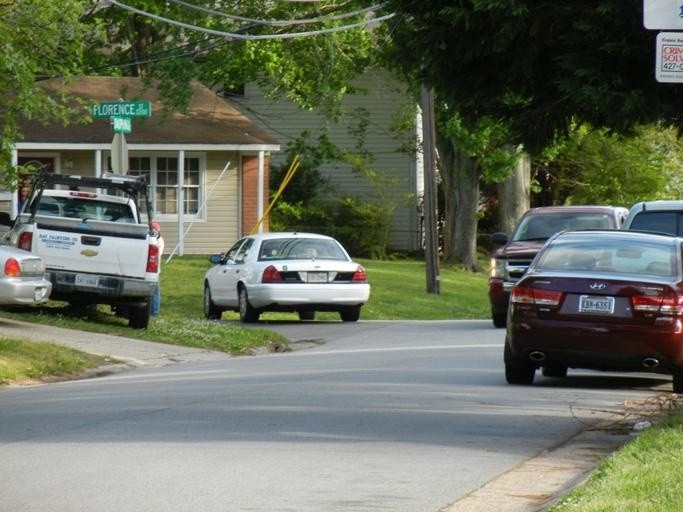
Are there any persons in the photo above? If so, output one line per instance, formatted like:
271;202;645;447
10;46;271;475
18;185;29;213
148;221;164;316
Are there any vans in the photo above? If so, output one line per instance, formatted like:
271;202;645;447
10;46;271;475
622;199;682;234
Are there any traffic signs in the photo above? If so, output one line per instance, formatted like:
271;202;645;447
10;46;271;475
88;101;150;117
112;118;132;133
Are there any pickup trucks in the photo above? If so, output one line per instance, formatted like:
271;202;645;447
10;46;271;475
0;169;160;329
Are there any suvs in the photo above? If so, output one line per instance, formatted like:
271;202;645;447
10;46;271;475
488;205;625;326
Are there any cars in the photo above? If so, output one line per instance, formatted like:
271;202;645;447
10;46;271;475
503;228;683;394
202;231;372;324
0;245;54;309
